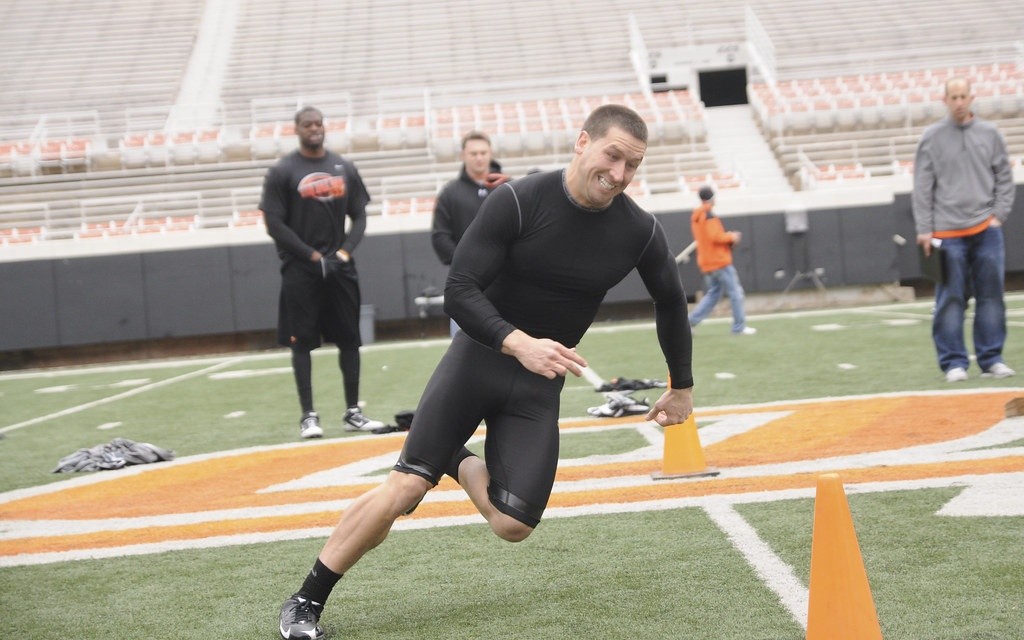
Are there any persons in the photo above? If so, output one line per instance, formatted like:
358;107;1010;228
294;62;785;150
783;166;809;192
279;104;695;640
432;130;512;336
687;183;755;334
259;106;386;440
914;76;1015;382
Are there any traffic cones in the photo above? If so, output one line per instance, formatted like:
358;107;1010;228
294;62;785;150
652;368;721;481
807;474;883;640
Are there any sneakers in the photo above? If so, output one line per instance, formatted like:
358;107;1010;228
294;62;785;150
299;411;322;438
980;363;1016;380
279;595;324;639
742;326;757;336
945;368;968;384
342;407;385;432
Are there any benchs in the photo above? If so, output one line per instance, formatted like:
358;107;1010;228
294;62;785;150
0;0;1024;271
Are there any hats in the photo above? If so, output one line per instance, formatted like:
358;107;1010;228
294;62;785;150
698;185;714;201
